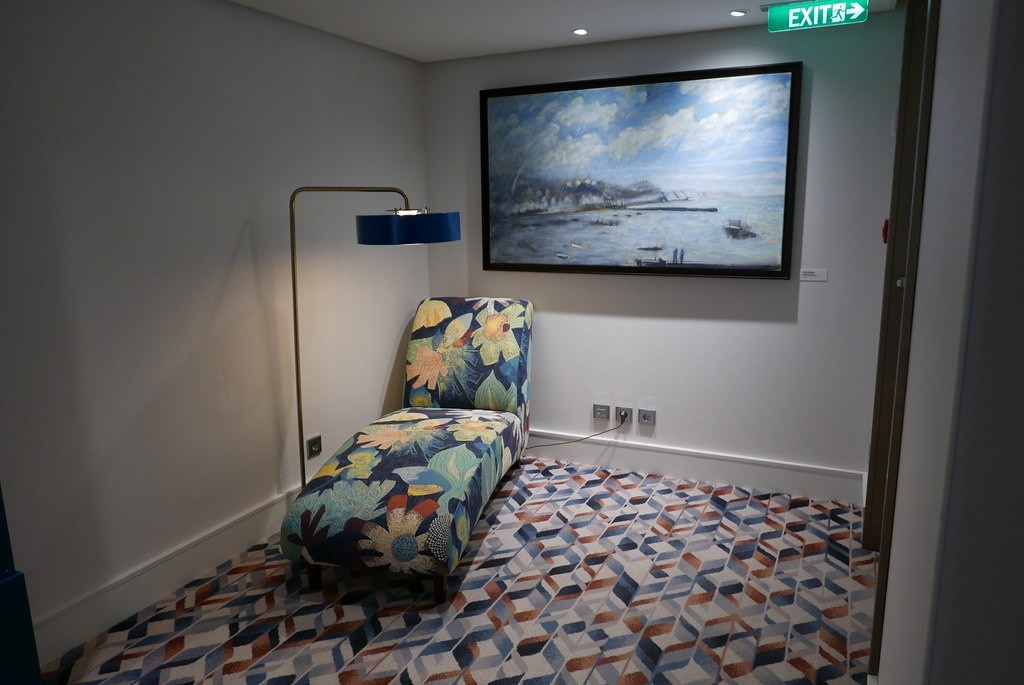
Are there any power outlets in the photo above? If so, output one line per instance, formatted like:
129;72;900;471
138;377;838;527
307;434;322;460
593;404;609;420
639;409;655;425
616;407;632;424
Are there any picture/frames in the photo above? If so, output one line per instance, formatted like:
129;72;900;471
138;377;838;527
479;61;804;280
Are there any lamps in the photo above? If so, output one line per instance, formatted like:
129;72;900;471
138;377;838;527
288;185;463;488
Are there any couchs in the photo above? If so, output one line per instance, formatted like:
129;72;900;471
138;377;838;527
280;298;534;606
